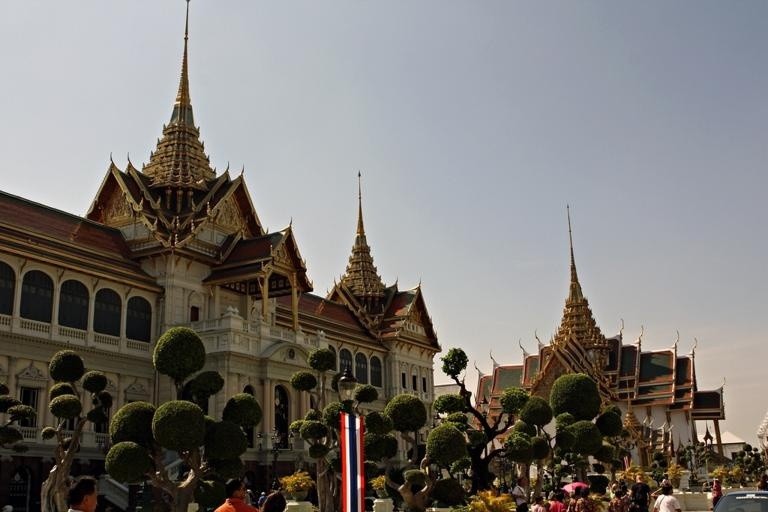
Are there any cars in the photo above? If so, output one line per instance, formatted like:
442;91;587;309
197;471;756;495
712;490;768;511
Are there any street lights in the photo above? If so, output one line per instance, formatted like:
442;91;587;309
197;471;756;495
480;394;489;426
257;427;294;481
337;367;359;413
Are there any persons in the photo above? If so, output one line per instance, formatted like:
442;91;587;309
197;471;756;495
759;473;768;490
212;477;262;511
259;491;287;511
711;474;722;505
510;472;683;512
257;490;268;507
63;476;98;512
